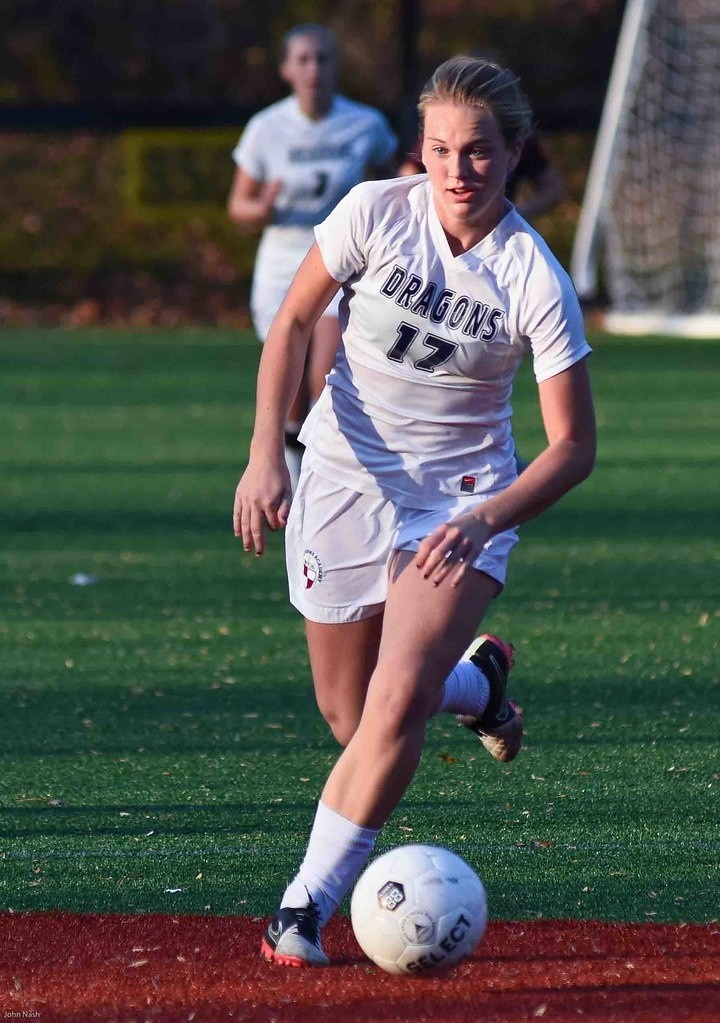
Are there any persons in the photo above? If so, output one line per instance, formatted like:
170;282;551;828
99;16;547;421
394;125;568;224
229;56;601;969
224;24;421;499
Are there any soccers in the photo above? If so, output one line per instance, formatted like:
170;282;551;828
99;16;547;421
349;841;490;978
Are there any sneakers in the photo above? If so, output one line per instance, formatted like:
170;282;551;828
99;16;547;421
456;634;521;762
260;884;329;968
